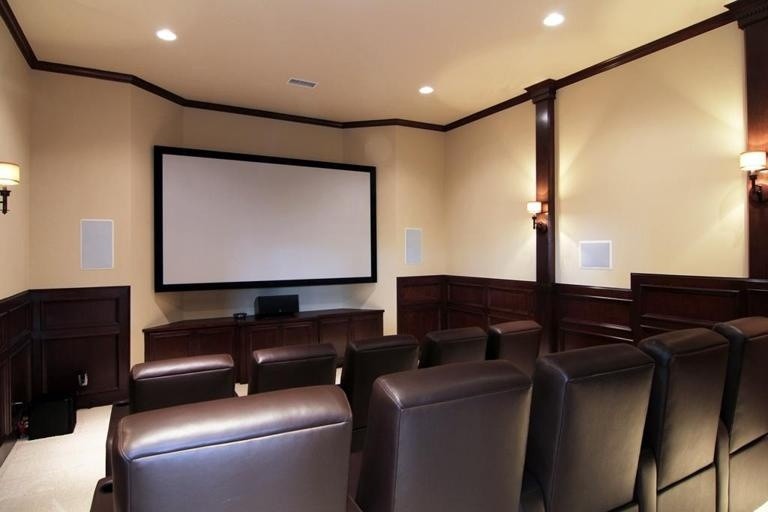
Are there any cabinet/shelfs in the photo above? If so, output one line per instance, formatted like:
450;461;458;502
319;308;385;369
238;310;320;384
141;317;238;384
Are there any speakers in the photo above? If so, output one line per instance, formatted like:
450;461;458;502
253;295;299;318
27;395;77;440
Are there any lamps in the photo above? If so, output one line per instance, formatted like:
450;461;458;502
738;150;767;204
0;160;22;216
527;199;552;232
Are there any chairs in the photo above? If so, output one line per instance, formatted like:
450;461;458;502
635;326;731;511
485;320;542;361
354;358;535;512
247;342;338;395
716;315;768;511
336;333;421;454
104;354;239;475
88;382;354;512
523;340;657;512
416;326;488;370
